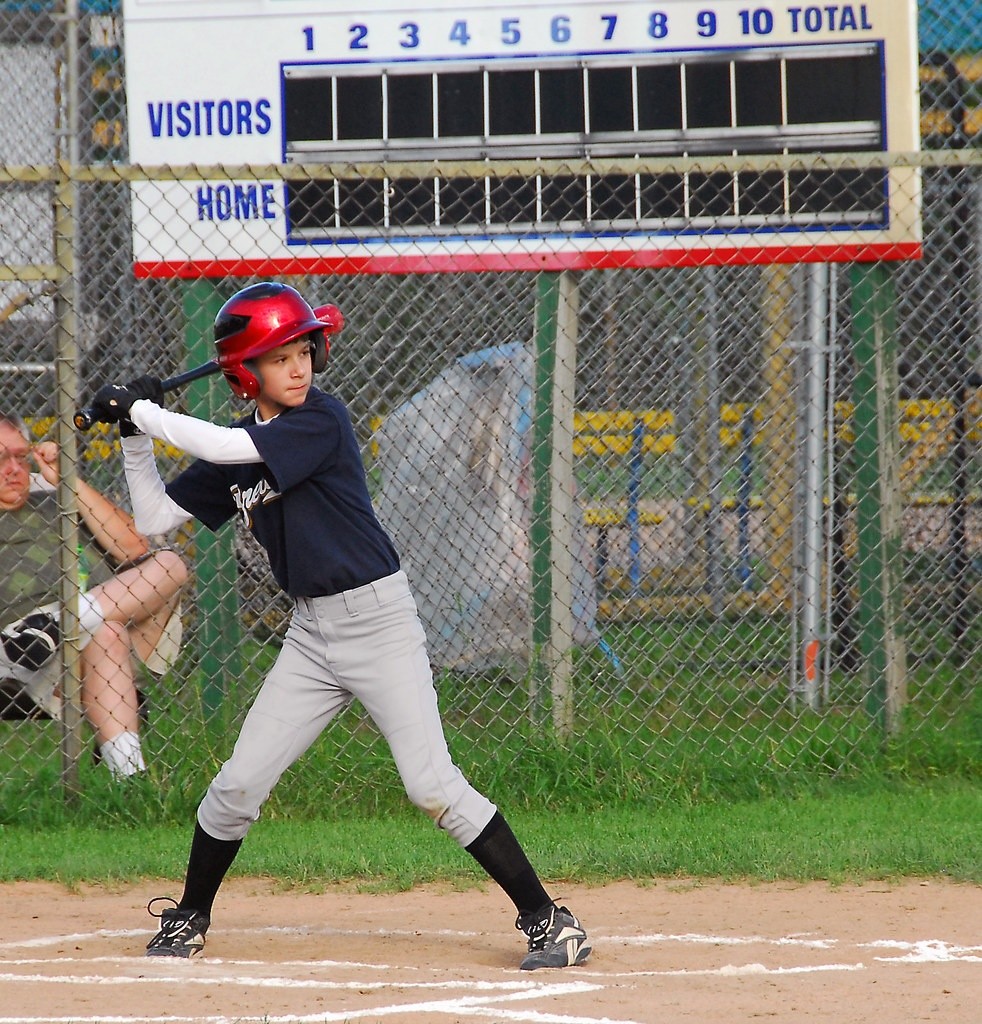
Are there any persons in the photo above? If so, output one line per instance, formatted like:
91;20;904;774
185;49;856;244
0;389;187;789
89;282;593;970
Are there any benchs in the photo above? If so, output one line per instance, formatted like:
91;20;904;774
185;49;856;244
24;391;982;605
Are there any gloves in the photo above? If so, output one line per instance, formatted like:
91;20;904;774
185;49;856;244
90;382;153;424
119;374;165;439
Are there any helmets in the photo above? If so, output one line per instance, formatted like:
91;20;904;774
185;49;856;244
213;282;334;400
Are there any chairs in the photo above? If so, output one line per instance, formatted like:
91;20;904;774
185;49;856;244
0;472;160;781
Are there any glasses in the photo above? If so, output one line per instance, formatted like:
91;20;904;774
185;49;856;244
0;444;38;464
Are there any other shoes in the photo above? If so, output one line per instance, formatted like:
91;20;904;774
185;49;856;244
1;613;59;671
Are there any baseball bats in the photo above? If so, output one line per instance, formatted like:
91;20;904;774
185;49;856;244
70;302;343;420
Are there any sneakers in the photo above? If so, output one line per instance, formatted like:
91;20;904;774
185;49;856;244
146;897;211;958
515;898;592;970
